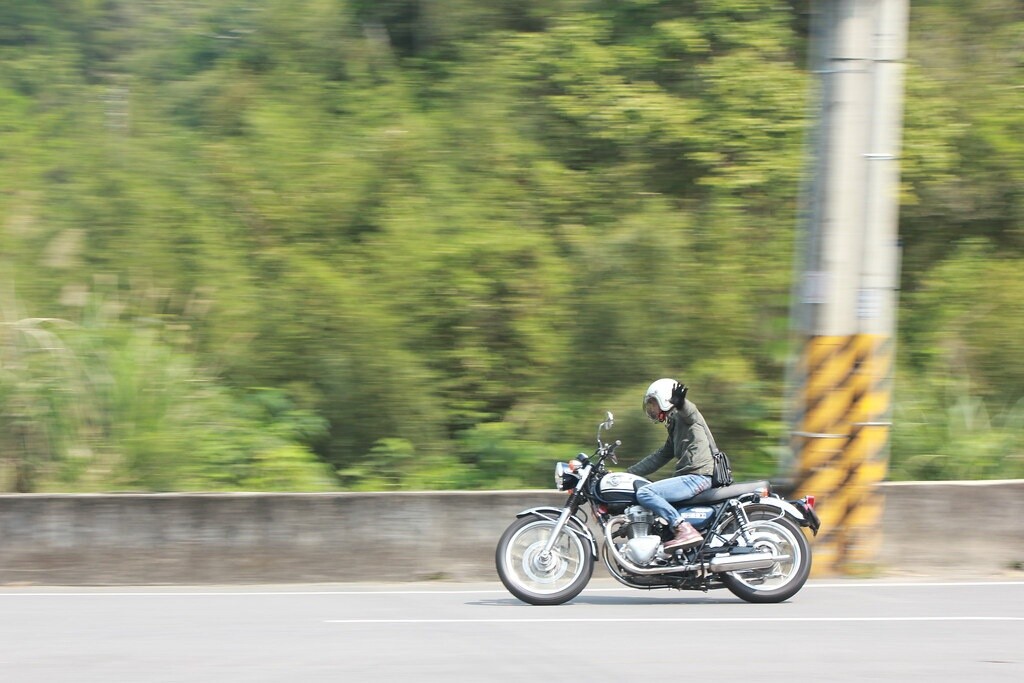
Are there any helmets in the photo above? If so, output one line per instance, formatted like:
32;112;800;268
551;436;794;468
643;378;685;424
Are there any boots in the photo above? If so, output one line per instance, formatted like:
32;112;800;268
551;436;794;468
663;522;705;554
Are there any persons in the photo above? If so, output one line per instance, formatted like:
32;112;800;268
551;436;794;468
626;378;718;550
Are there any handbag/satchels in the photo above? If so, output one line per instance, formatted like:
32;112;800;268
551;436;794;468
712;451;734;488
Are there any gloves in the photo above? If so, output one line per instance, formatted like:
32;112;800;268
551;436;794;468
664;381;689;412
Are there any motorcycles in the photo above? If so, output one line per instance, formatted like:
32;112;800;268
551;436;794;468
495;411;820;605
